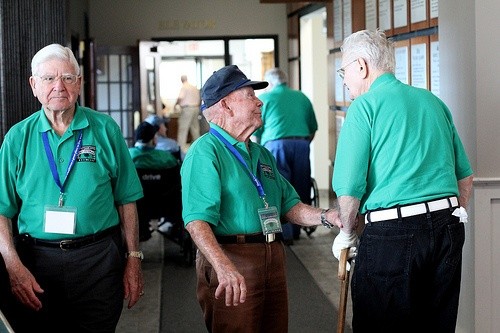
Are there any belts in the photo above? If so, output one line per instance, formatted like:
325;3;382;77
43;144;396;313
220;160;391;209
364;196;460;224
16;225;120;252
217;233;283;245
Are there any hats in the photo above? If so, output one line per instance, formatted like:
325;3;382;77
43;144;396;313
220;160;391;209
144;114;172;126
199;64;270;111
134;121;159;143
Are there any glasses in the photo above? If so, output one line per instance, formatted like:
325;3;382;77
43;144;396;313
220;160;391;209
34;72;81;85
337;58;359;79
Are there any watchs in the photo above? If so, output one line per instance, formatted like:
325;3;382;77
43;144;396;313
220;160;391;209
321;208;334;229
125;249;144;261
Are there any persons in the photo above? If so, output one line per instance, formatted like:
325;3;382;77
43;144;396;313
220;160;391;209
174;72;203;151
179;65;361;333
328;27;472;333
123;120;187;233
248;68;319;246
144;115;186;159
0;44;147;333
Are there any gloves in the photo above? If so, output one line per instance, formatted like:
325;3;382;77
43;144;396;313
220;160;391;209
331;228;361;272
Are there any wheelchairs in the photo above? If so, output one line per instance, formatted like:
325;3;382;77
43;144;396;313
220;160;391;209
124;165;197;266
